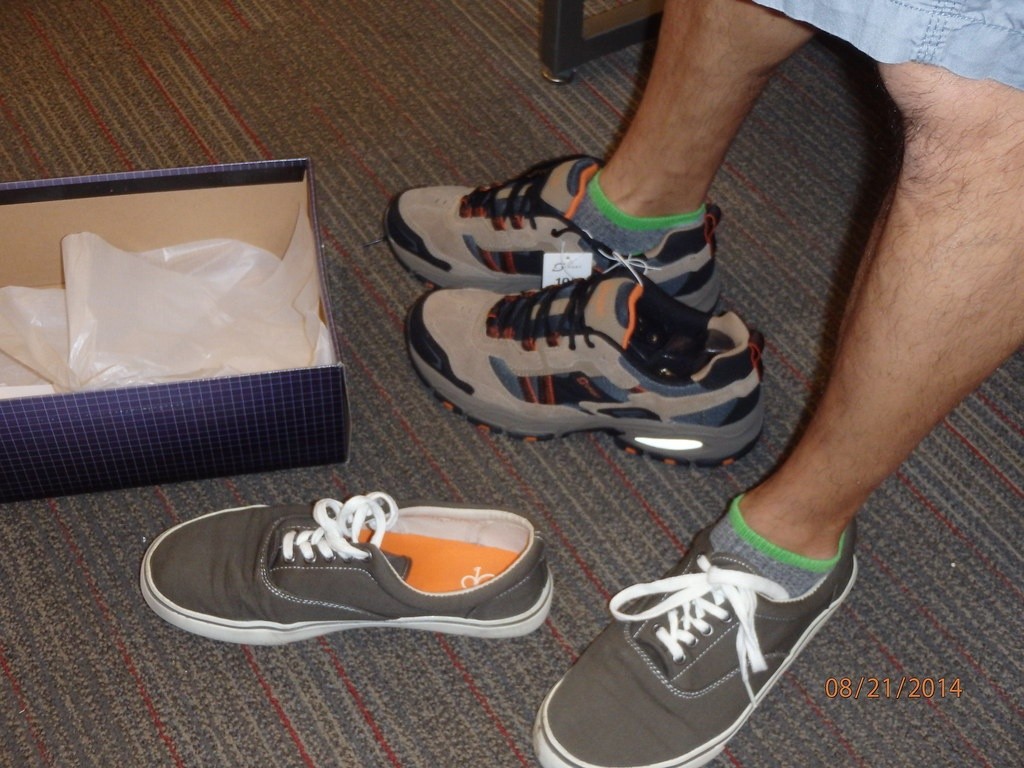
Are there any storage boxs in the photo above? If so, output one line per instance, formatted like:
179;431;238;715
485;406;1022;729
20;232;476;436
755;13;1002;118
0;154;351;500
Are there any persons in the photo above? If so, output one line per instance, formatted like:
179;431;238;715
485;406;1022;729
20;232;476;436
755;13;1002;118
384;0;1024;768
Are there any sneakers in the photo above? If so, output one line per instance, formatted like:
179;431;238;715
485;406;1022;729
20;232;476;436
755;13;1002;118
404;275;767;468
136;488;554;647
528;520;857;768
381;153;723;317
